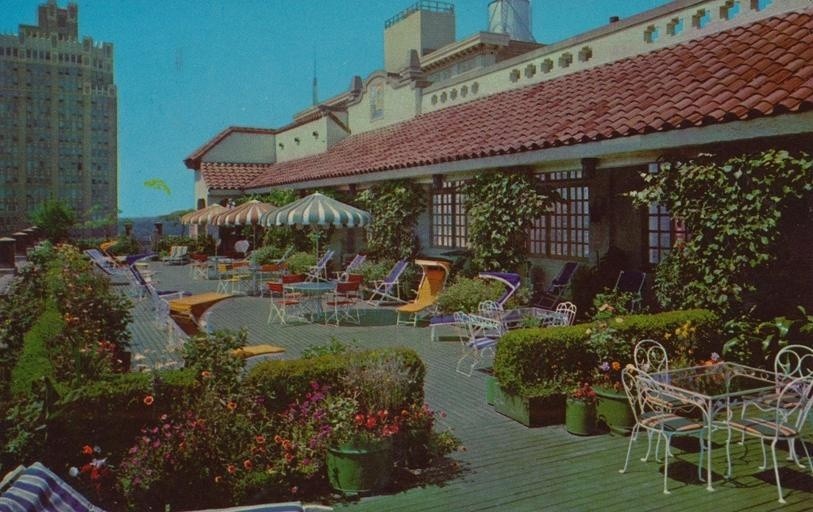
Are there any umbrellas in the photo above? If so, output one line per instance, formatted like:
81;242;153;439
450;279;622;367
204;197;279;294
258;189;375;319
178;201;230;275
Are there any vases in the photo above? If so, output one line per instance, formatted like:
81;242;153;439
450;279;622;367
592;382;647;434
564;400;598;436
324;440;397;496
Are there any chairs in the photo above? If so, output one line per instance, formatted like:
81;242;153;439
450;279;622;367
599;269;647;317
618;341;813;507
396;249;579;381
0;460;302;511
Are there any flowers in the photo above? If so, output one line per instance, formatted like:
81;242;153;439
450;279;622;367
568;382;598;404
310;401;397;447
591;359;650;391
